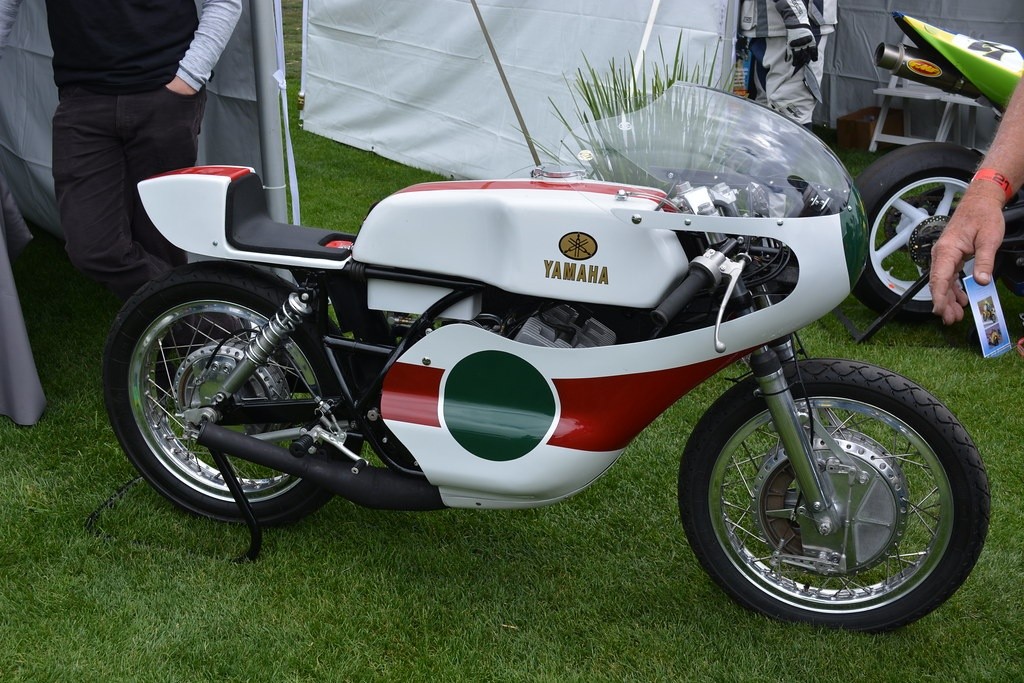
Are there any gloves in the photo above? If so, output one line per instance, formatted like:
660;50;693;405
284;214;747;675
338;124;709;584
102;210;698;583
785;24;818;67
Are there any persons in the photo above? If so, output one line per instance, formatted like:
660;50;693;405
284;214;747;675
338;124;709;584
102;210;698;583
737;0;838;126
984;301;991;312
930;72;1024;324
990;329;996;339
0;0;246;389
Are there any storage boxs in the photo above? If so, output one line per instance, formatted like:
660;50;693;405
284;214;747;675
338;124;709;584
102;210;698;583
837;106;903;151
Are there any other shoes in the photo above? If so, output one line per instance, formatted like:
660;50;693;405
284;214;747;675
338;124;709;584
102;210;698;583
239;318;251;337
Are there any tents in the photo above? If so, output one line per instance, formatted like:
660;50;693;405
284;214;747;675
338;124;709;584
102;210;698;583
297;0;1024;181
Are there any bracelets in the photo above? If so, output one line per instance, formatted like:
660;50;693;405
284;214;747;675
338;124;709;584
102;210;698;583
971;169;1013;204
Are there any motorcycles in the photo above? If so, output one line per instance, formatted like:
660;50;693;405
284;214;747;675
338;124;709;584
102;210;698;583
833;9;1024;345
98;80;991;631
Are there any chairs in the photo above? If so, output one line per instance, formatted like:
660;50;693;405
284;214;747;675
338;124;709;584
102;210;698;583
868;34;1001;154
